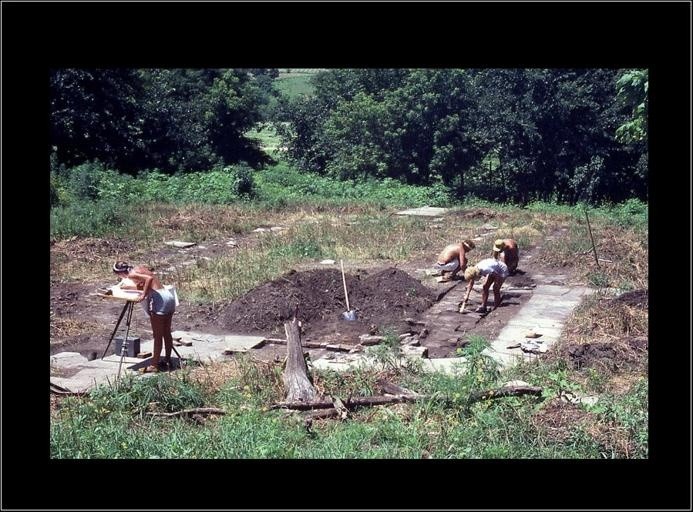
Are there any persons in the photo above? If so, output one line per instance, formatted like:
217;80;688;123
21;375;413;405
111;261;176;373
492;237;518;276
462;257;509;313
434;239;475;281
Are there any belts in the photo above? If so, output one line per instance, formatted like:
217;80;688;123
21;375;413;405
438;263;447;266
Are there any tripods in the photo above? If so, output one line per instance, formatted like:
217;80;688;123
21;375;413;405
101;300;181;380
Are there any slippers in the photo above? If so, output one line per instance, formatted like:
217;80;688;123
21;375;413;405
138;367;160;374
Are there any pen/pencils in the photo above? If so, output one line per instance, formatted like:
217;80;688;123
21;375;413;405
120;282;124;286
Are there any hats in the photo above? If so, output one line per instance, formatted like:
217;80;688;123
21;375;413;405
492;239;503;252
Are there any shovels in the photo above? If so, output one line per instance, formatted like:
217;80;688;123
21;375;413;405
340;259;357;322
